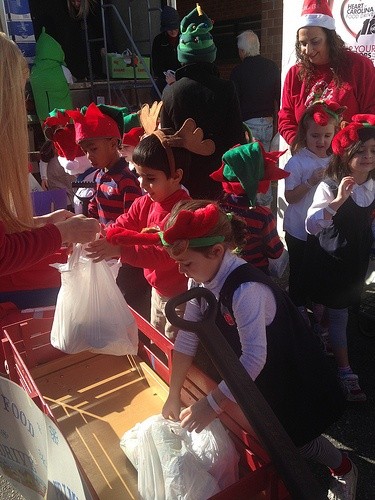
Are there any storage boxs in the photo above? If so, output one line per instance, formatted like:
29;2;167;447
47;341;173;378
105;53;151;78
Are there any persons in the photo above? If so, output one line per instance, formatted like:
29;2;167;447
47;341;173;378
29;26;74;139
303;115;375;401
159;5;247;201
208;140;290;275
283;101;341;328
157;199;359;500
229;28;281;210
149;5;182;101
85;126;207;375
67;0;110;80
0;32;101;271
278;0;375;152
38;103;150;223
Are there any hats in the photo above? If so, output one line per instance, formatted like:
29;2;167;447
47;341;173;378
208;140;291;210
296;0;336;32
176;3;217;64
43;102;146;176
159;5;180;33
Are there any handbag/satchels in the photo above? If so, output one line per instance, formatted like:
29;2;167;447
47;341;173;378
50;230;139;356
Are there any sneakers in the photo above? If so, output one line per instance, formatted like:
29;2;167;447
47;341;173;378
335;373;366;401
313;327;335;357
299;303;313;328
328;451;358;500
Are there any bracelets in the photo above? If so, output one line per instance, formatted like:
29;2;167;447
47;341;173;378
206;392;224;415
325;205;337;216
305;179;314;189
